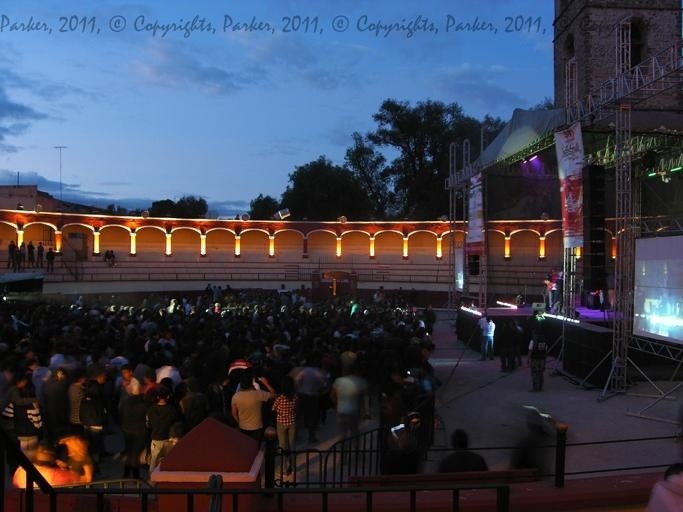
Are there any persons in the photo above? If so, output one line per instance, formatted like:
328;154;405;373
479;314;549;393
104;250;115;265
438;427;489;472
644;462;682;512
1;283;437;489
7;239;58;274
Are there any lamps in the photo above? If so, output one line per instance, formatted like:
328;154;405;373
649;173;657;177
10;224;626;262
669;167;682;173
528;154;538;162
523;160;528;164
